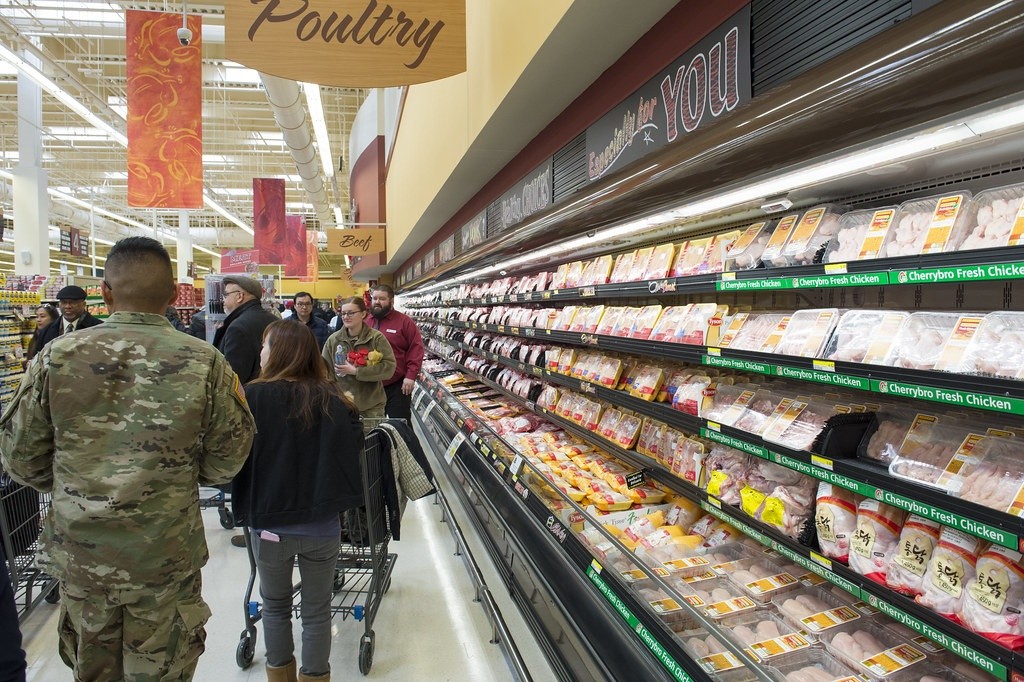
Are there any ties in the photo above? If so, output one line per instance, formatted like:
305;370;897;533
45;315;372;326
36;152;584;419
66;323;74;333
374;317;381;330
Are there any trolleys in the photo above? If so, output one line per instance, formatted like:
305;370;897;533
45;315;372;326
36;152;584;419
0;466;61;624
236;417;407;674
198;482;235;529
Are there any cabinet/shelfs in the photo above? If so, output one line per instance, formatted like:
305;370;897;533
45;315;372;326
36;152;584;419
392;1;1023;682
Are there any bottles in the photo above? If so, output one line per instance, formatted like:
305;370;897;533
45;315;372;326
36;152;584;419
335;344;344;369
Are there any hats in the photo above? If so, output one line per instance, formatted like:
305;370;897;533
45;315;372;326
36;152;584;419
56;285;86;301
222;275;262;299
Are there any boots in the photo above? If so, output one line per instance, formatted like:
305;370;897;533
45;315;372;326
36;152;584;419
298;665;330;682
265;654;298;682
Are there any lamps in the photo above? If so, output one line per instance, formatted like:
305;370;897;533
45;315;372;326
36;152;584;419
0;0;351;273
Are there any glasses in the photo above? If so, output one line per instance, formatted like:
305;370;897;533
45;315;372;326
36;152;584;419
296;303;313;307
222;290;244;297
339;310;364;318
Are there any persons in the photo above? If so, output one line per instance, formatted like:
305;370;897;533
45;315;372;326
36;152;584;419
213;276;278;386
364;284;424;432
322;296;396;432
266;293;343;355
228;319;365;682
0;235;256;682
166;305;206;340
22;285;104;372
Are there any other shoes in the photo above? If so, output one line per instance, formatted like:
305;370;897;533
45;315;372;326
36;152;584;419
231;532;247;547
